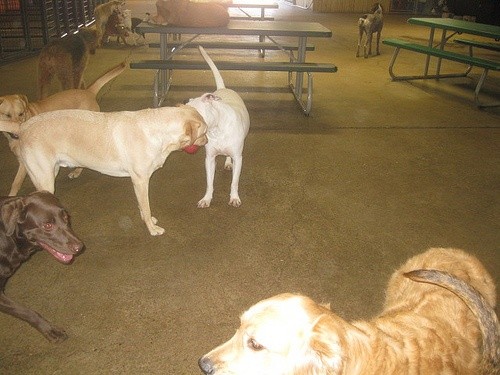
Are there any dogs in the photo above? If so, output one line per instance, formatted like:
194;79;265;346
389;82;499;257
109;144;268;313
0;103;211;238
37;29;100;101
356;3;386;58
93;1;152;48
0;189;85;344
196;246;498;374
185;45;249;210
0;64;126;196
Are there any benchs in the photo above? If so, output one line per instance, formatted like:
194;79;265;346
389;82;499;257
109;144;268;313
383;37;500;111
131;17;338;113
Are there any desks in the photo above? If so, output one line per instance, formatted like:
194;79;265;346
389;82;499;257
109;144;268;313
408;18;500;75
138;21;331;99
229;4;279;57
